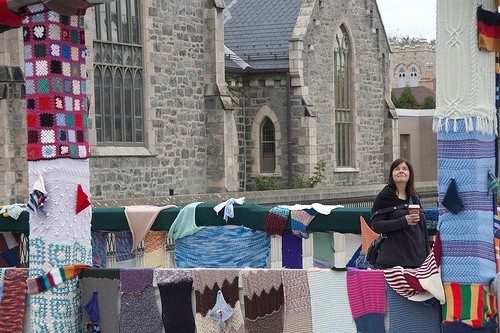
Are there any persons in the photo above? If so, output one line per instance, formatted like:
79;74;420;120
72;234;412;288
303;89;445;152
371;159;431;270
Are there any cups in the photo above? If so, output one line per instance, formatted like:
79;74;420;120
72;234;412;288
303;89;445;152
408;204;420;223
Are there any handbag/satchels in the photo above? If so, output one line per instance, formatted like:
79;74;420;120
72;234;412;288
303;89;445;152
367;234;385;264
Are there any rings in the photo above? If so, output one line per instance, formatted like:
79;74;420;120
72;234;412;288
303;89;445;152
411;219;414;222
